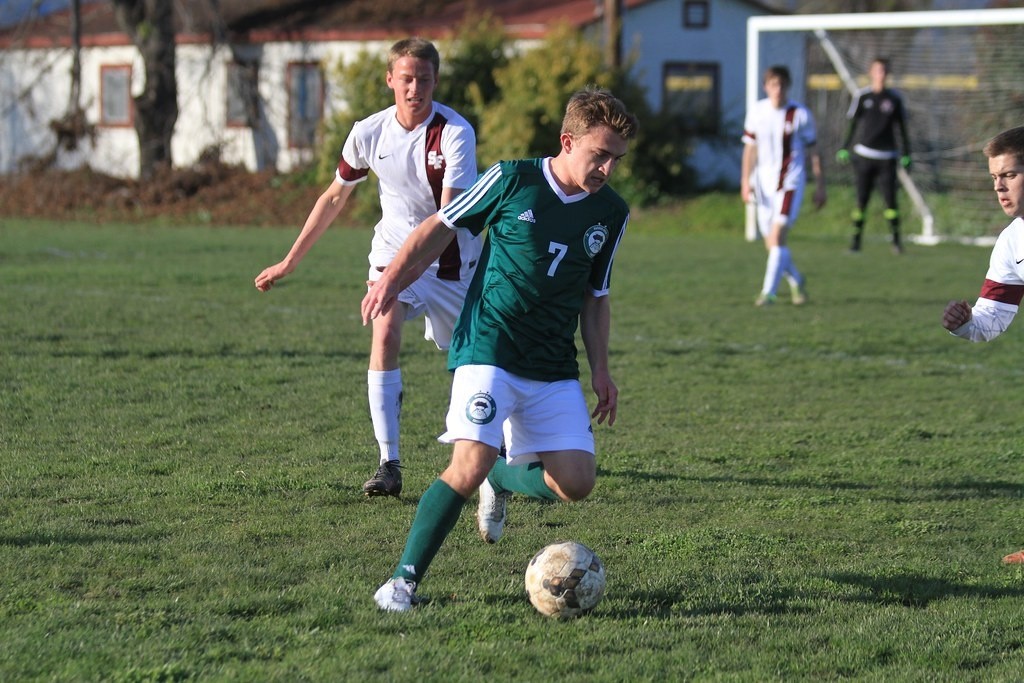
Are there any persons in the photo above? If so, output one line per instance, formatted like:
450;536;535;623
361;89;639;612
741;65;827;306
835;58;913;254
942;127;1024;564
253;38;506;498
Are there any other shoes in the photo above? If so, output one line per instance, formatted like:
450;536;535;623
891;239;903;254
1004;550;1024;564
850;241;859;252
757;294;776;306
790;277;807;305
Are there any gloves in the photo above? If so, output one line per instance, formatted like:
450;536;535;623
836;149;850;165
900;156;911;175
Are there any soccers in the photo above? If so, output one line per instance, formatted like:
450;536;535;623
524;541;605;617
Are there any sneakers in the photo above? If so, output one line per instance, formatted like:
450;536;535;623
477;454;507;544
374;576;420;611
363;459;408;498
